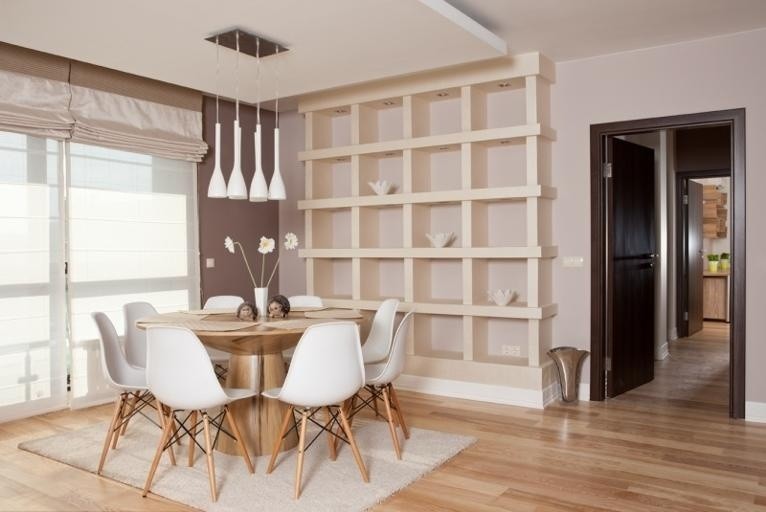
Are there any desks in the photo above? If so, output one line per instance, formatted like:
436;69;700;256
138;308;363;457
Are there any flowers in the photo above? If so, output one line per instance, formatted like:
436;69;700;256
223;232;300;288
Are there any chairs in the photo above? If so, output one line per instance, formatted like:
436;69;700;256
334;297;414;459
142;324;254;503
261;320;370;498
93;301;191;474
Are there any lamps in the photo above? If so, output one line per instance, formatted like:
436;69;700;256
207;29;287;203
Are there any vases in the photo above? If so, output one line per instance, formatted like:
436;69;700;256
254;287;268;318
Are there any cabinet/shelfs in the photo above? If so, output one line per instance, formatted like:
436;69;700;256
703;269;731;322
295;51;561;389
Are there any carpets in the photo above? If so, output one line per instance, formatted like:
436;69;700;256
17;406;484;512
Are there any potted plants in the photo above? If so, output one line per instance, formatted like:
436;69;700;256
720;252;729;270
707;254;718;273
